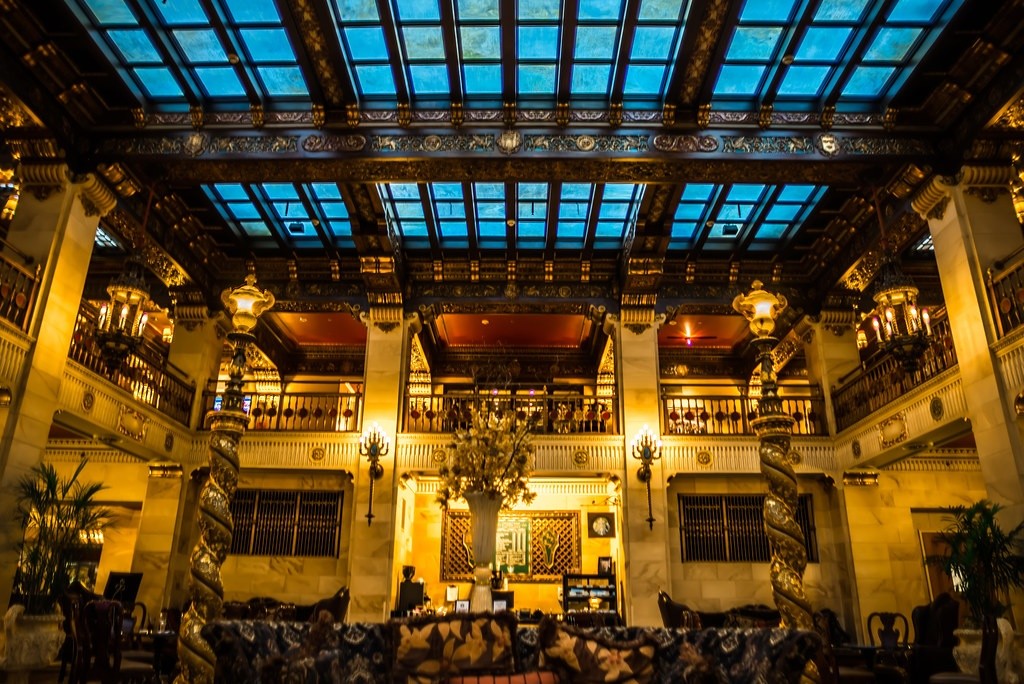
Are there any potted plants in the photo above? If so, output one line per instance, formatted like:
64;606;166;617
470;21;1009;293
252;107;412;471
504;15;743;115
925;498;1024;678
9;457;118;667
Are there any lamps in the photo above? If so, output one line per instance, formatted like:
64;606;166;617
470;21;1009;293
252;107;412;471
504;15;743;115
732;277;827;684
630;426;666;530
722;224;739;235
289;223;303;233
172;264;276;684
359;426;390;527
92;190;156;376
866;182;932;373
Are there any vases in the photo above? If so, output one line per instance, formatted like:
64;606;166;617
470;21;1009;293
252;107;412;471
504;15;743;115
461;490;502;610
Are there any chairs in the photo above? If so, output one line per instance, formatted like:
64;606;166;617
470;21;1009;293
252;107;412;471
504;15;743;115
70;601;151;684
866;612;911;675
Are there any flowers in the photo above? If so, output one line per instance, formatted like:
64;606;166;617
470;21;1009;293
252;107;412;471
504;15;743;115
438;406;539;508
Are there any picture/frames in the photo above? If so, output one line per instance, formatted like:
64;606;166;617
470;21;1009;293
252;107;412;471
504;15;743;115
916;528;1015;630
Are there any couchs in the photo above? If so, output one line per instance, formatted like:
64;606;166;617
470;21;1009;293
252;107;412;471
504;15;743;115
198;617;823;684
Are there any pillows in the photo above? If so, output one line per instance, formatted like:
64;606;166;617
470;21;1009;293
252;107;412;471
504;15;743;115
390;618;520;684
536;616;661;684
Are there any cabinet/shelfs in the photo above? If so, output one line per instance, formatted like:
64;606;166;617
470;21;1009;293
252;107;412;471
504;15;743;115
560;572;619;615
